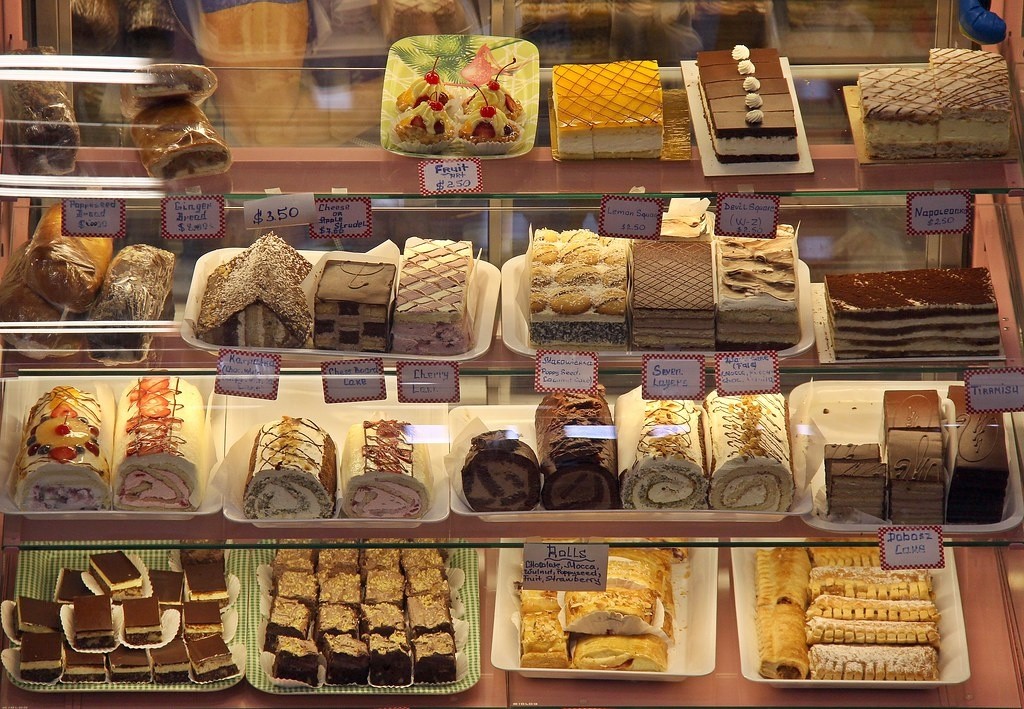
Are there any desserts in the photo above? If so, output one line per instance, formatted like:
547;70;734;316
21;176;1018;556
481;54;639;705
0;40;1019;688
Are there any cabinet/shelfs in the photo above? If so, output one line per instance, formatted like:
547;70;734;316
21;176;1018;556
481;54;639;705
0;0;1024;709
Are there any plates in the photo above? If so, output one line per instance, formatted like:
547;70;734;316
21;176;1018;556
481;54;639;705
500;253;815;362
248;537;482;694
681;56;815;177
490;537;719;681
844;85;1020;167
2;376;224;519
447;403;790;521
7;536;250;691
811;283;1009;364
730;536;972;689
180;246;502;366
222;375;452;526
380;34;541;159
545;87;691;162
790;380;1022;531
